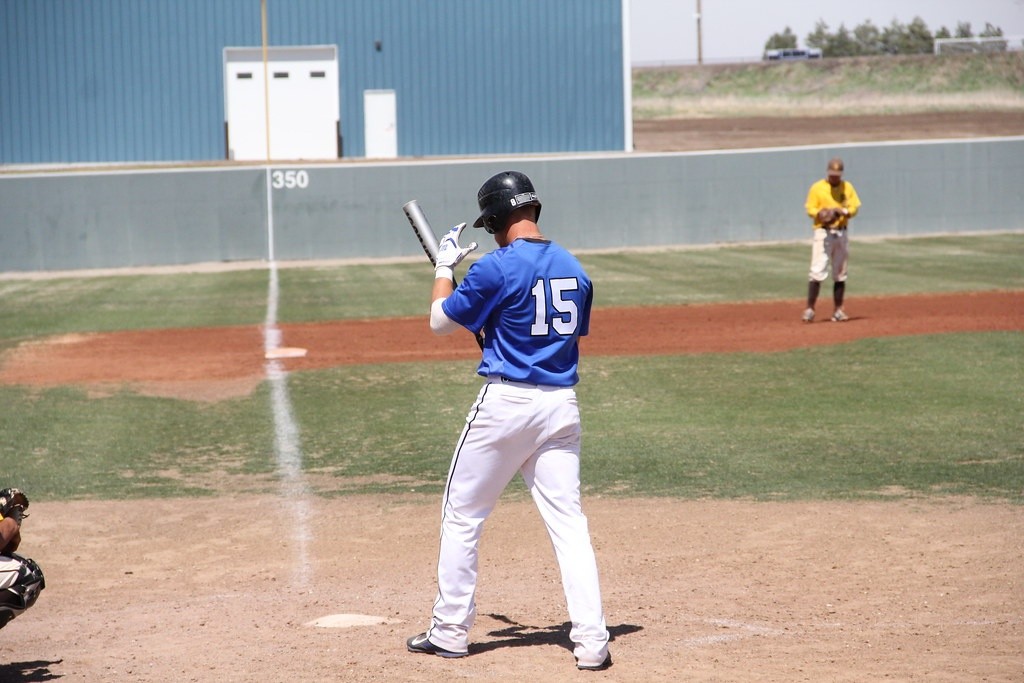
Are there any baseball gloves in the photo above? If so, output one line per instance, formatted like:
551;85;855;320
0;487;30;553
818;207;842;226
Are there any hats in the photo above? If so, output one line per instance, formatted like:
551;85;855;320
827;159;845;176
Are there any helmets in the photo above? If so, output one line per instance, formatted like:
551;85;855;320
473;171;542;234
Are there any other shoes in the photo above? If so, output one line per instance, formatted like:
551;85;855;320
830;310;849;322
803;308;814;320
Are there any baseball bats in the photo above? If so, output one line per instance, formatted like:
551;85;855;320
403;198;484;353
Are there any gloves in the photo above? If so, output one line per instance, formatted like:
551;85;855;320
434;222;478;271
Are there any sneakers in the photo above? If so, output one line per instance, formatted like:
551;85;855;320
577;651;612;670
406;632;469;658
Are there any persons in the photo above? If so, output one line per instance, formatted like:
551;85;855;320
0;487;46;632
803;159;861;322
407;171;610;671
